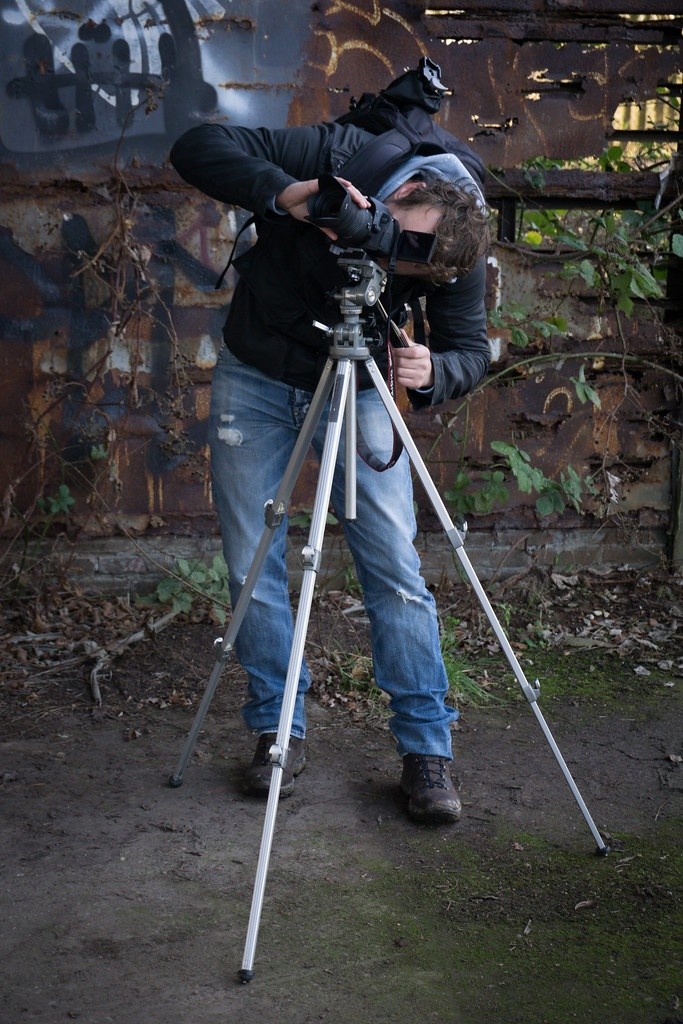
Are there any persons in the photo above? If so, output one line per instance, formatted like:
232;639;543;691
171;120;494;822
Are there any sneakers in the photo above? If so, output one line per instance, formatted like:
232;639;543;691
399;752;463;824
242;732;308;798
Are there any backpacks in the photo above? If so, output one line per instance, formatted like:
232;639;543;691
214;96;490;293
